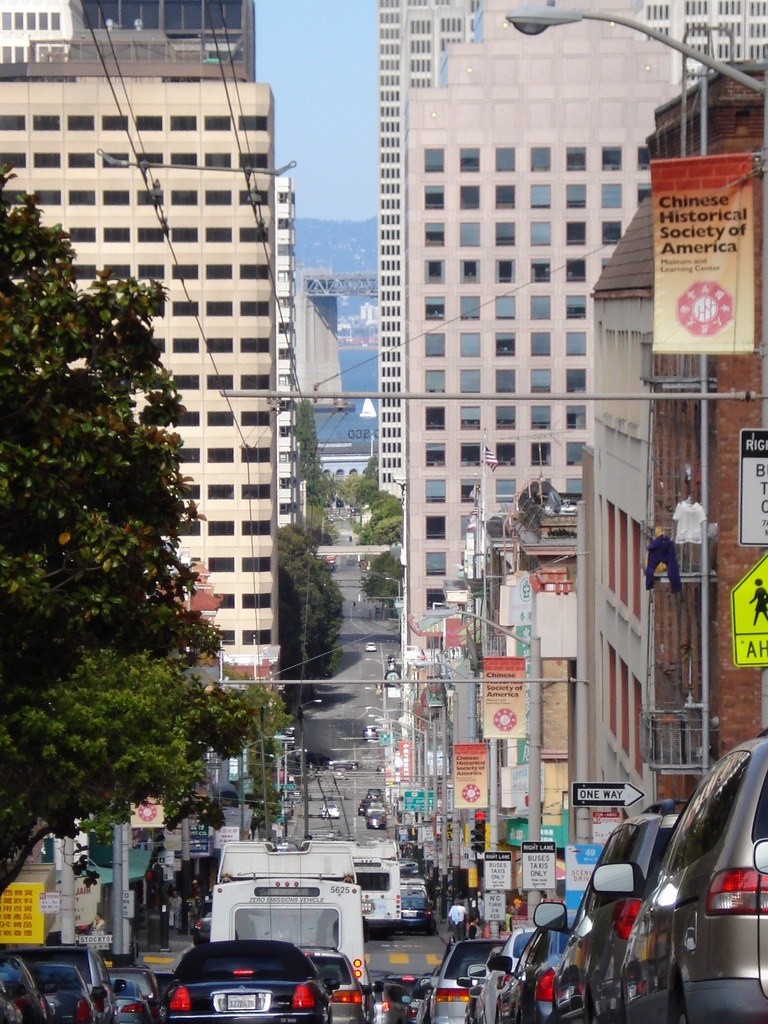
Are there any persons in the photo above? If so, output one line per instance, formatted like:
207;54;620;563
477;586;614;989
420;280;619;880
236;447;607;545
191;879;200;900
446;883;528;943
168;889;182;929
88;912;106;936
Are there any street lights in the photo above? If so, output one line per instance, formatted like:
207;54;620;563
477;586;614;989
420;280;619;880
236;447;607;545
300;699;322;708
240;609;541;928
506;7;768;97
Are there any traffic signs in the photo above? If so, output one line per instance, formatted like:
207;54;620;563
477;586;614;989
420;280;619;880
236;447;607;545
570;781;647;808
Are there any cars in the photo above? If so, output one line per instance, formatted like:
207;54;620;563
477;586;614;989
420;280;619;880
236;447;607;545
0;718;768;1024
365;642;377;652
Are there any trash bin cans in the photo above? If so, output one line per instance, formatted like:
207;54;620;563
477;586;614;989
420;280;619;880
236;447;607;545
147;910;169;952
